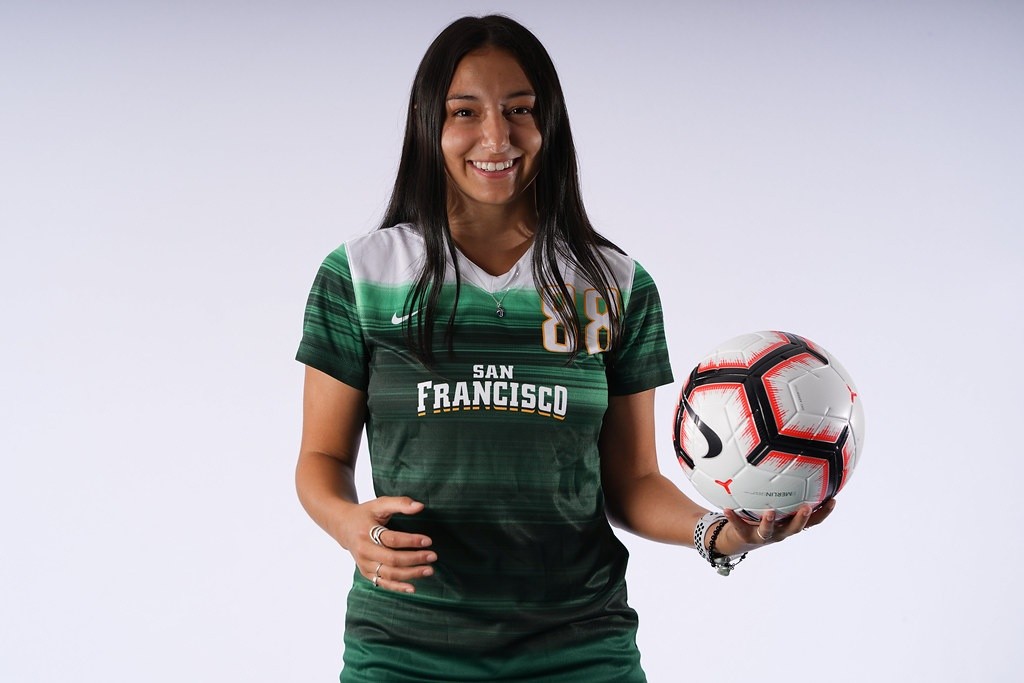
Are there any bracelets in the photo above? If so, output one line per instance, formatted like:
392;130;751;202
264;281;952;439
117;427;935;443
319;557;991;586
693;512;748;576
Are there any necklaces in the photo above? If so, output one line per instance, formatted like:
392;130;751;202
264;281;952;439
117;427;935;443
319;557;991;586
451;238;535;317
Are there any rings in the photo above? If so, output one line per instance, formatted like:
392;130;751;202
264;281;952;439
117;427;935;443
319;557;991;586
369;524;389;545
757;527;774;540
375;563;383;577
373;576;381;587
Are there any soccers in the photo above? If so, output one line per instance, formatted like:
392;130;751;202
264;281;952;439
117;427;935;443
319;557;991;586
672;332;872;524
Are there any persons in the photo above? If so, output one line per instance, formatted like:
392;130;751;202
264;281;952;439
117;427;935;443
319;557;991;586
294;14;836;683
